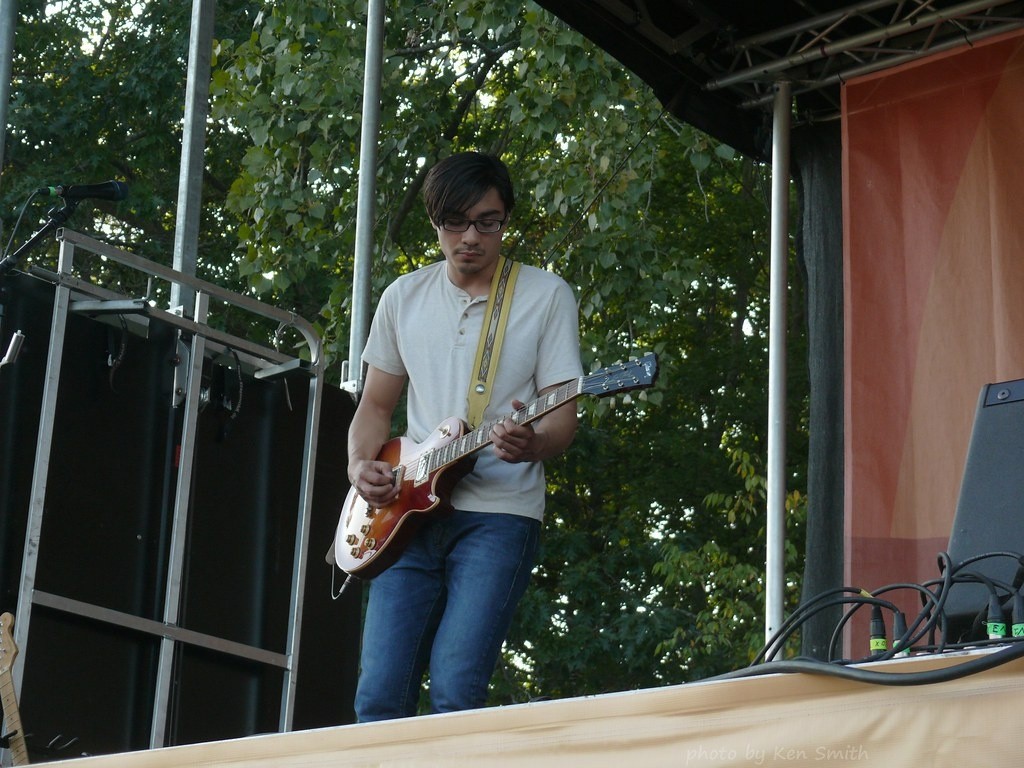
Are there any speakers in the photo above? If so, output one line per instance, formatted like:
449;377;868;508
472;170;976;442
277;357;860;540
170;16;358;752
939;378;1024;648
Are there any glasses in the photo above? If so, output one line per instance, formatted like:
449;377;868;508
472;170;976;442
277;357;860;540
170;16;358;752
443;211;508;234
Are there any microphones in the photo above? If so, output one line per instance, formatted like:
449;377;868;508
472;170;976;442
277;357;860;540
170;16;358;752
38;181;128;201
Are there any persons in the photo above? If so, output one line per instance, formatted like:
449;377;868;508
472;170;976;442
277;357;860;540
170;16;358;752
347;154;584;723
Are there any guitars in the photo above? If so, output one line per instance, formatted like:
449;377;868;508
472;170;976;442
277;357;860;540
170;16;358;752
334;352;661;581
